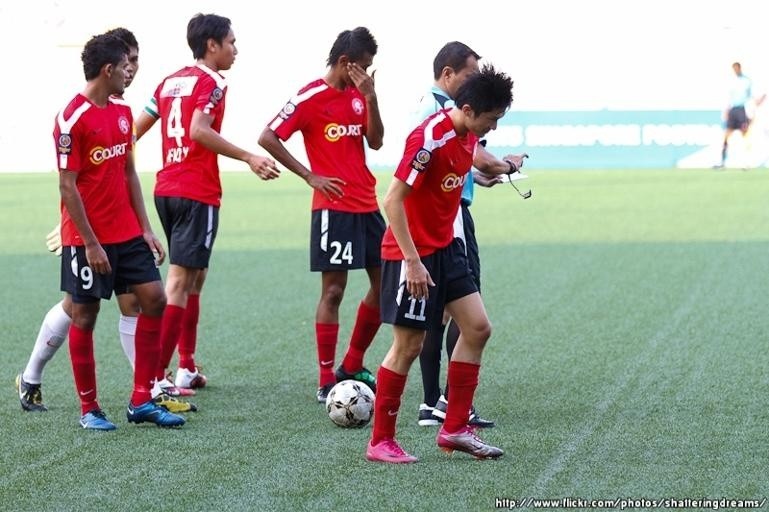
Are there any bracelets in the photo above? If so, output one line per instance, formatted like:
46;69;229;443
502;158;518;174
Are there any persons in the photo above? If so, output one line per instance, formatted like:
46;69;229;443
713;61;766;172
365;63;515;463
132;14;282;395
256;28;387;405
413;39;529;430
52;33;191;433
13;27;199;422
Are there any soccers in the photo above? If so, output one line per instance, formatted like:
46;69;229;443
327;380;376;429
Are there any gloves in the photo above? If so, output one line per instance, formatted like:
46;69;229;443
46;225;62;256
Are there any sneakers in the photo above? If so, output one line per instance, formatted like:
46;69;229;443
159;371;195;396
437;425;504;460
432;395;496;427
151;391;197;413
16;373;47;412
336;365;377;394
175;367;207;388
418;404;443;426
127;400;184;429
80;409;117;430
367;437;417;463
316;383;336;403
